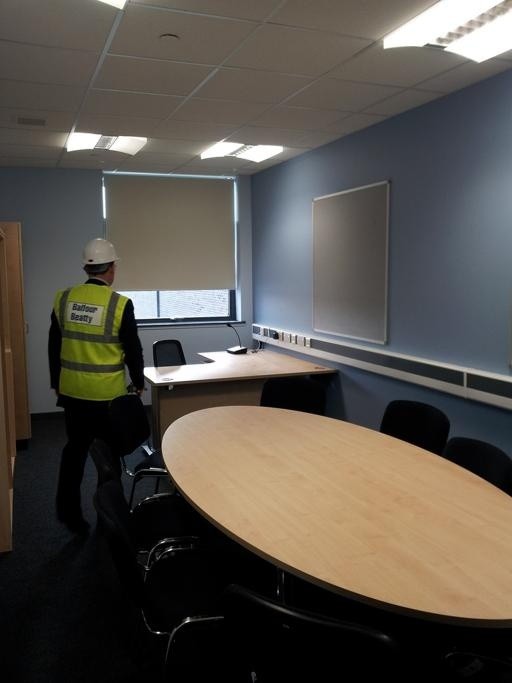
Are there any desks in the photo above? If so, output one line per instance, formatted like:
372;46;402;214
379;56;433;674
143;348;338;449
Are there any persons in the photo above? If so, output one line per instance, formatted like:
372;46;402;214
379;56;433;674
47;235;147;526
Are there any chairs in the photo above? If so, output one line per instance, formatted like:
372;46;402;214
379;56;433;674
152;338;186;367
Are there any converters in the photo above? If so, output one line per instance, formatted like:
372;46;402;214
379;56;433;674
273;333;277;338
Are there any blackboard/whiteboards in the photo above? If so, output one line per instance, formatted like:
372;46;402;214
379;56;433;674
311;179;390;345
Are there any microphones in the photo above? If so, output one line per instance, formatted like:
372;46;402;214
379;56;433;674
226;322;247;354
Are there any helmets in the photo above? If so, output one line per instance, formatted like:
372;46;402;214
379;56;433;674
81;238;120;266
